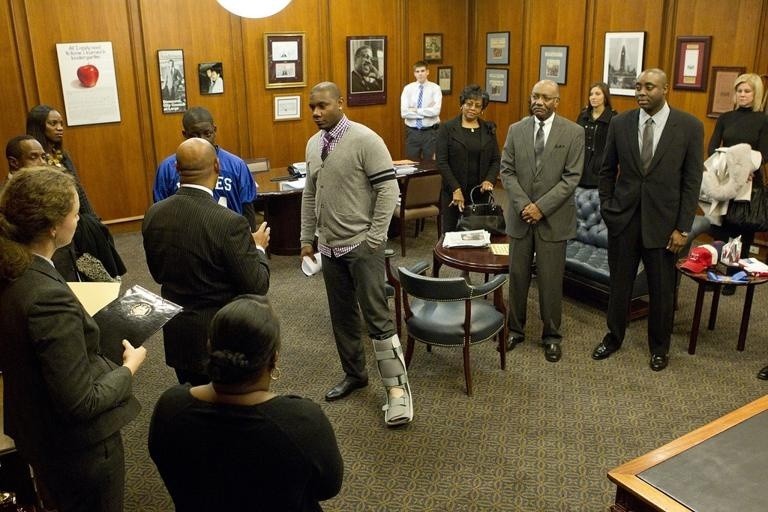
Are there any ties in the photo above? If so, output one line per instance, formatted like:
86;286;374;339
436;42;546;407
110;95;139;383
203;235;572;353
533;120;545;172
640;118;654;176
415;85;424;130
320;133;334;161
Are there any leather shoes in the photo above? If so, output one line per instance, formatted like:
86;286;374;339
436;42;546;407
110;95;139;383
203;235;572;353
496;333;527;352
758;365;768;381
591;343;611;359
649;352;670;372
324;373;372;400
544;343;562;363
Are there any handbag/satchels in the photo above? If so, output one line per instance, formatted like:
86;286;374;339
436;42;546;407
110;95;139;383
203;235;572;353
456;185;507;236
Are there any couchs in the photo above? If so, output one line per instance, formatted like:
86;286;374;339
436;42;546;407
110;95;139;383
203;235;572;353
532;186;711;328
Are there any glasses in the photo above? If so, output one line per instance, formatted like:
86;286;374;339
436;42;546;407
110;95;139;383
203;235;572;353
532;94;558;101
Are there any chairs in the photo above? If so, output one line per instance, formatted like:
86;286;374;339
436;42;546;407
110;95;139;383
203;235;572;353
244;156;270;212
385;249;432;340
394;171;445;257
397;260;509;396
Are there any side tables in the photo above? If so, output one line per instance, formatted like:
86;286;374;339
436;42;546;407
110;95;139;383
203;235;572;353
671;255;768;355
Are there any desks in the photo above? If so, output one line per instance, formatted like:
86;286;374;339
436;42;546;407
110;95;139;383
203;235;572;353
251;159;440;256
606;393;768;512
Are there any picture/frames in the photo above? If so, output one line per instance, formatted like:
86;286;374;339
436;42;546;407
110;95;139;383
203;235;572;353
485;68;509;104
437;66;453;95
602;31;647;98
272;92;304;122
538;44;569;86
423;32;444;64
706;66;746;119
486;31;511;66
261;30;308;89
157;48;188;114
55;40;122;127
345;35;388;107
672;35;713;93
198;62;225;95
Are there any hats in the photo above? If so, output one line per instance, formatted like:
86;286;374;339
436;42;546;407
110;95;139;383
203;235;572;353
680;244;718;273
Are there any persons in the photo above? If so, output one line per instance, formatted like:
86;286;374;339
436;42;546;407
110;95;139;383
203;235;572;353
0;167;146;512
25;105;90;214
162;59;183;100
6;134;79;282
141;137;270;385
496;78;585;363
592;67;705;371
576;82;619;190
152;108;258;233
400;62;442;160
435;84;501;233
205;68;223;94
352;46;382;92
705;72;768;297
300;81;414;427
148;293;344;512
